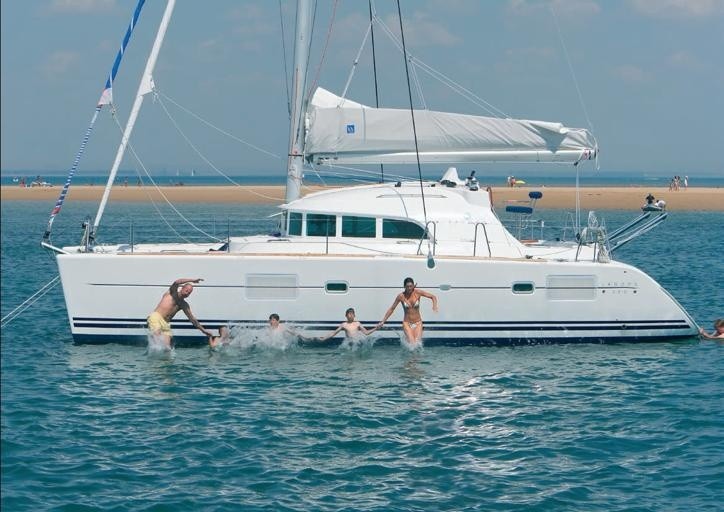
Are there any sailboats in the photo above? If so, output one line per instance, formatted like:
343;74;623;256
41;0;701;343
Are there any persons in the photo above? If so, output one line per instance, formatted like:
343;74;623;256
12;175;41;188
507;175;511;187
124;176;129;187
209;325;231;353
645;193;655;207
656;200;666;211
669;174;689;191
376;277;439;346
265;313;312;348
698;319;724;340
317;308;382;351
470;170;477;181
511;176;516;187
145;277;214;355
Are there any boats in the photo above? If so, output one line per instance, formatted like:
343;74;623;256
643;204;663;211
